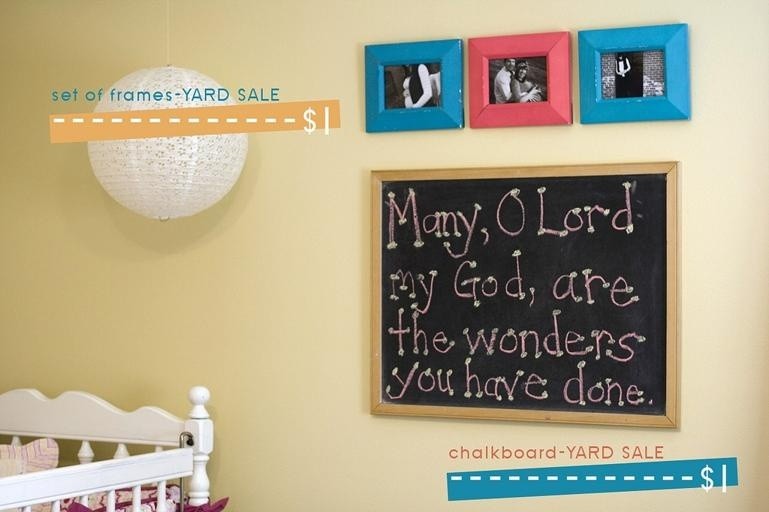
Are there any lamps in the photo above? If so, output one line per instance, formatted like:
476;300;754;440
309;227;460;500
87;2;247;222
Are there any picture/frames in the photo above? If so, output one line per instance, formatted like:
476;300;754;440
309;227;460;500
363;38;465;132
578;22;692;124
467;31;573;128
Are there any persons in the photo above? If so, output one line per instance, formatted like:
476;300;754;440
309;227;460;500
401;63;434;108
492;58;539;103
614;52;634;98
510;60;541;103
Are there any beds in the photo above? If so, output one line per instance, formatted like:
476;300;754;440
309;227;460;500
2;385;215;512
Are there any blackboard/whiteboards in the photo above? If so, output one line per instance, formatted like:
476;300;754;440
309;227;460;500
369;160;683;429
